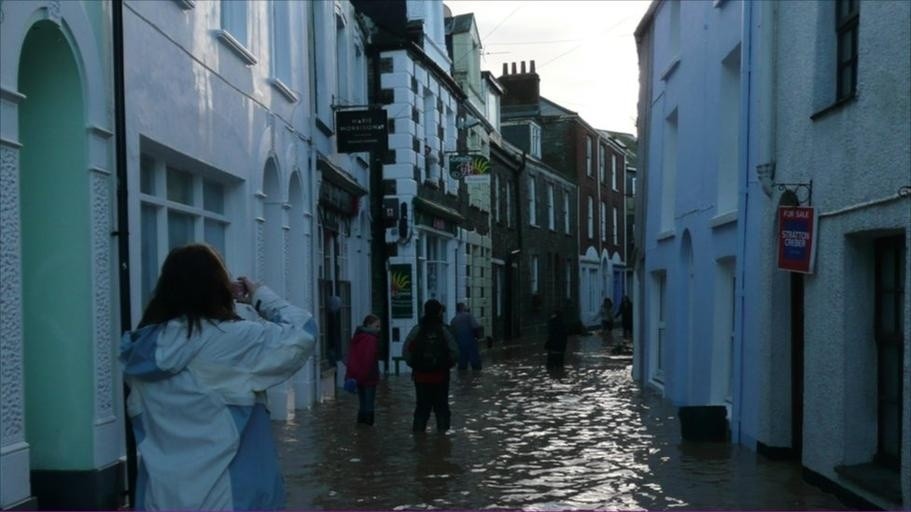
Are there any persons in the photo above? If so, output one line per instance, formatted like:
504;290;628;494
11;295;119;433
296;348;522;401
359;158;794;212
591;296;616;332
538;296;593;370
114;241;320;511
451;302;486;371
614;293;633;332
401;297;462;430
344;314;384;426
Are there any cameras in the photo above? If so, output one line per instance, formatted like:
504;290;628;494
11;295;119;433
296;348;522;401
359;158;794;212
229;281;248;298
